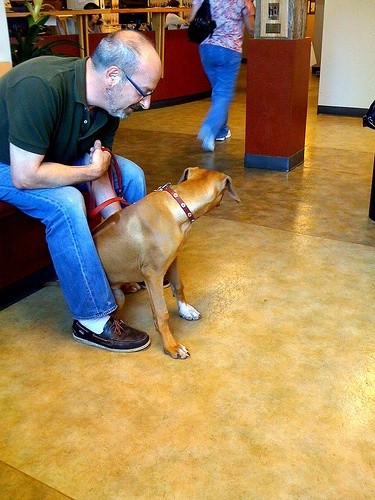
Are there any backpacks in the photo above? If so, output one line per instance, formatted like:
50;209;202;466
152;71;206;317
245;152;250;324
187;0;217;44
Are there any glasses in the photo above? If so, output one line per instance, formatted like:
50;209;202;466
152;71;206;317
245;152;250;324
124;72;154;100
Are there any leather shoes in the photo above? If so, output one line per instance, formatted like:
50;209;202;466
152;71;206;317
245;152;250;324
72;316;151;353
139;279;171;288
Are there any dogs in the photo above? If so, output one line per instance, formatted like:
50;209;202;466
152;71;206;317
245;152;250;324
92;166;241;360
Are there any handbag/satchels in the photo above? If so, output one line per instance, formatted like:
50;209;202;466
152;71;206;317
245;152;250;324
363;100;375;129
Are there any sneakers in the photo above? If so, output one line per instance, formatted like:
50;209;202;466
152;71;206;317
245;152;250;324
198;126;215;152
216;128;232;140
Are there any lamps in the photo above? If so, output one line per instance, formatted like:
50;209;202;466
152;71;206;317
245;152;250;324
307;0;316;15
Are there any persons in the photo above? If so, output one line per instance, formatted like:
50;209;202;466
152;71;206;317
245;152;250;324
38;0;185;34
0;30;172;352
190;0;256;152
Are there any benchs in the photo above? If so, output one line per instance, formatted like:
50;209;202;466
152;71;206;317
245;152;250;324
0;184;102;291
